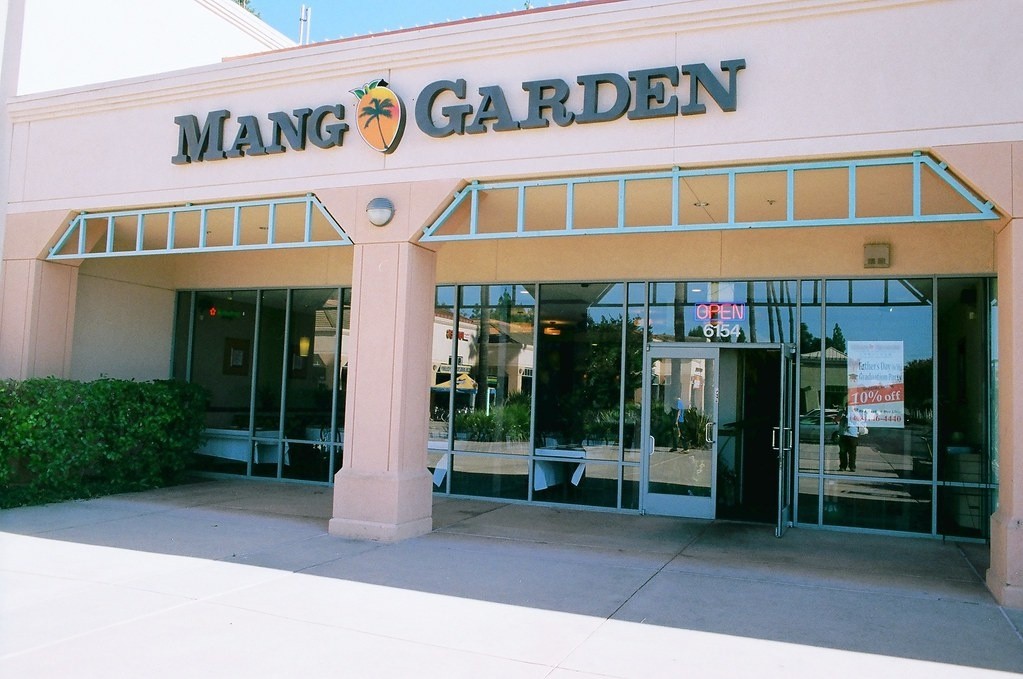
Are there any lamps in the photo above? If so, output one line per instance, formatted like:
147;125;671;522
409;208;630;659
365;197;394;227
299;335;313;356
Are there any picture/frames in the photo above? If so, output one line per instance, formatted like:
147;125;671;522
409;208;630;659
222;338;251;376
287;342;309;379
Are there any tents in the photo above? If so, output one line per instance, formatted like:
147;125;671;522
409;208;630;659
431;373;497;413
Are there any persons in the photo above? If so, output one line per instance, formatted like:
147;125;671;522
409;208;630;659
834;401;863;472
669;391;691;454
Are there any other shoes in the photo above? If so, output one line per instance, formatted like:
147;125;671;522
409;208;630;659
838;467;846;471
679;449;689;454
850;468;855;472
668;448;677;452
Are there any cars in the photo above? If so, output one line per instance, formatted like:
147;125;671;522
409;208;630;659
799;408;869;444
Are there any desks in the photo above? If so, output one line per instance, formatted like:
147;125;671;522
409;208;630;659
534;445;587;490
304;427;343;452
196;427;289;466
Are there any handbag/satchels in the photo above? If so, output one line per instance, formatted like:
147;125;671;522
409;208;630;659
859;424;868;436
672;424;681;438
831;430;840;444
